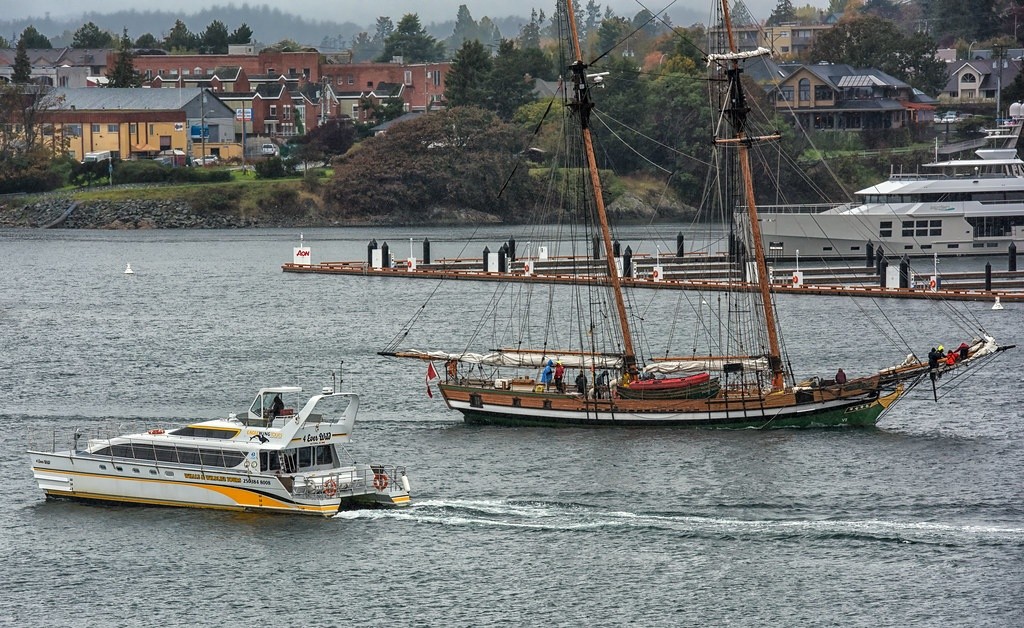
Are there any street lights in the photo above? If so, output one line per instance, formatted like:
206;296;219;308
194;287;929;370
968;41;978;60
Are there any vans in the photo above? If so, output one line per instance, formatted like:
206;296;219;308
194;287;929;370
260;143;277;156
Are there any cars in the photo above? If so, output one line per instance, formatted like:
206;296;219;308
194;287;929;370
933;111;974;123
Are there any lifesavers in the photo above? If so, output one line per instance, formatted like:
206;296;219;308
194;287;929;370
525;265;529;271
373;474;388;490
653;271;658;277
148;428;166;434
793;275;798;283
930;280;935;288
323;479;337;496
408;261;411;267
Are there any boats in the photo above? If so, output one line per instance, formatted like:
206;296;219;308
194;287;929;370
733;102;1024;261
25;362;413;516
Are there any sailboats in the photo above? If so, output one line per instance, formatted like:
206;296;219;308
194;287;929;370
377;0;1018;430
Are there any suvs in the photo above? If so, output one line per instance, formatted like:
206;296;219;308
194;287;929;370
192;155;218;167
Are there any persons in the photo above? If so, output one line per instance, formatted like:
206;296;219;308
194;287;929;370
540;360;608;400
273;396;284;418
835;368;846;384
928;343;970;369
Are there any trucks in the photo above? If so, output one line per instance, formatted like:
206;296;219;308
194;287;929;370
85;150;111;162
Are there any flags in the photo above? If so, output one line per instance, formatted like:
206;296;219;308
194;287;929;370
425;376;433;398
428;362;437;380
